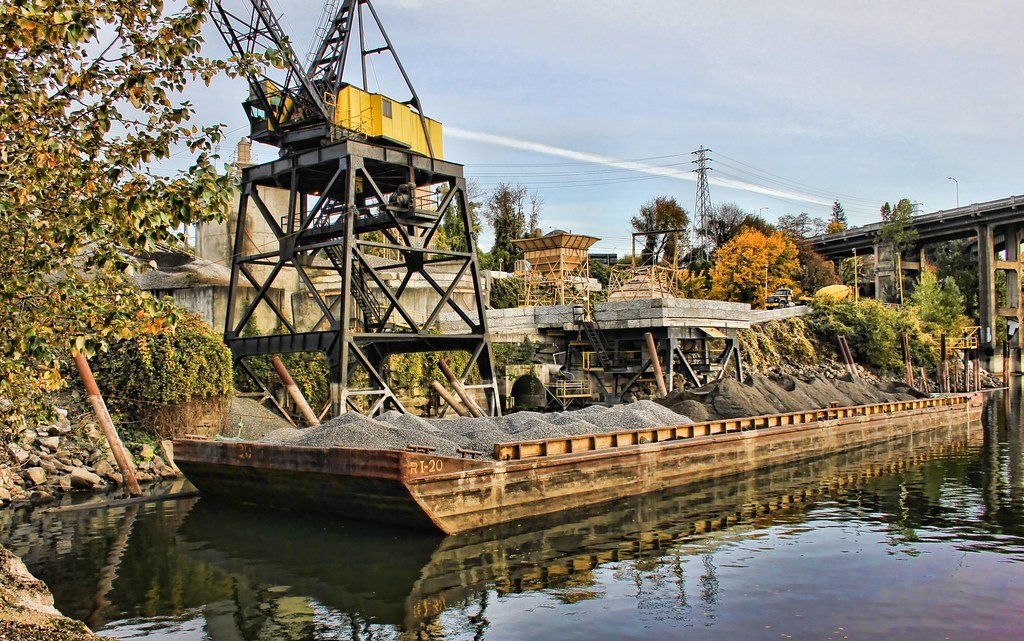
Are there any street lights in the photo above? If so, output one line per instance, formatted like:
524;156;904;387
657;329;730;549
948;177;958;208
760;207;769;220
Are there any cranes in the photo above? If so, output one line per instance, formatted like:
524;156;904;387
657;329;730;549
203;0;504;421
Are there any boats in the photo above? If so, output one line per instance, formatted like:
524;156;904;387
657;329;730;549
169;376;987;538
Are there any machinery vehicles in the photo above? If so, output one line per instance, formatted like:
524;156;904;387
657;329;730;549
765;286;814;310
